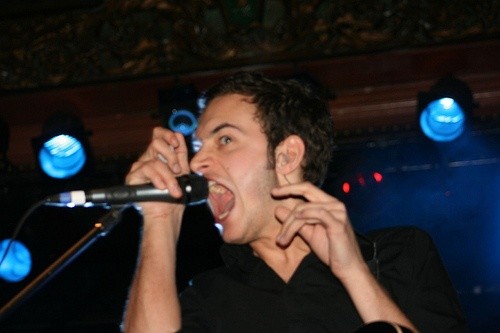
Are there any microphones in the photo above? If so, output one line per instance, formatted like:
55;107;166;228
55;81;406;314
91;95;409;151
48;174;209;208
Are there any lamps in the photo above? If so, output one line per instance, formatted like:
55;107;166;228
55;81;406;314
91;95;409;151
29;117;95;184
157;82;205;142
1;225;48;287
412;79;475;142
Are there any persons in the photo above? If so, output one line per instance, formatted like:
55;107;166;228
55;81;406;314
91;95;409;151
126;70;461;332
